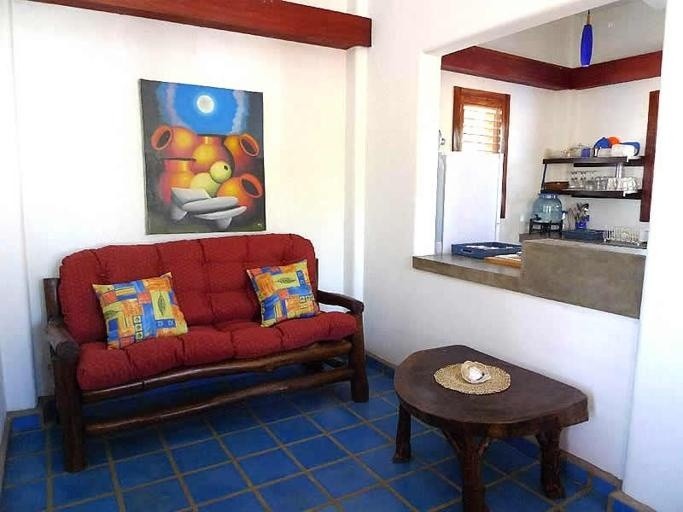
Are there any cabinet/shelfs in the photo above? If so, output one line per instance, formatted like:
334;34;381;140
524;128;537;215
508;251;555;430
540;156;643;200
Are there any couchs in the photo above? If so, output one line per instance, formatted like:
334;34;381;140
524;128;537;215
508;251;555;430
42;234;368;470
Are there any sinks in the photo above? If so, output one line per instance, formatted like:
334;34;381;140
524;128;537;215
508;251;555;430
562;228;611;241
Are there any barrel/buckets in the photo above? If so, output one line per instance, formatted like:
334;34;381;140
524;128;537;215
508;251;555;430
534;193;563;229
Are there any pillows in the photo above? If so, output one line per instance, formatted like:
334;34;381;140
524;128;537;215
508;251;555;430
92;273;191;351
245;259;321;327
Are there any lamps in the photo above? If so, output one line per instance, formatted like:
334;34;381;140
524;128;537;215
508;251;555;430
579;9;592;67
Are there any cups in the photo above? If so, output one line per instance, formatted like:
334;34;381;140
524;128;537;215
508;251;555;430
603;225;641;245
571;171;637;192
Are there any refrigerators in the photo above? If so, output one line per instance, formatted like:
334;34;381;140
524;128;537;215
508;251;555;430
435;151;504;255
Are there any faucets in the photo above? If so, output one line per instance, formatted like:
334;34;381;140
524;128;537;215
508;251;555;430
579;204;589;214
